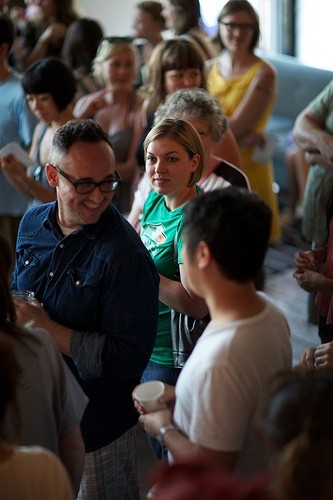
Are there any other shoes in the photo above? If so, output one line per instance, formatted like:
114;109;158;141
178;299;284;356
295;204;304;218
279;212;296;229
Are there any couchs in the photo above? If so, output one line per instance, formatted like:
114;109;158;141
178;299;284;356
264;57;333;204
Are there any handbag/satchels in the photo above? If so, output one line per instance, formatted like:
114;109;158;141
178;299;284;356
171;308;211;368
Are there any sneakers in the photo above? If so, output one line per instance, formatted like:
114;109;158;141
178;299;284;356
282;229;303;246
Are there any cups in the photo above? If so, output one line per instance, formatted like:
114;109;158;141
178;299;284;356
12;289;36;304
133;380;168;414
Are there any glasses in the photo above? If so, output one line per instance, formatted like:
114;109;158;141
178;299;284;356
99;37;133;47
54;166;122;194
220;21;255;31
33;165;42;184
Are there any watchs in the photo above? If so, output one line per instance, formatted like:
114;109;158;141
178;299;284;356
32;164;43;183
156;423;175;448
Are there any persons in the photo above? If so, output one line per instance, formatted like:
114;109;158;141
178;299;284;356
0;0;333;500
11;120;161;500
